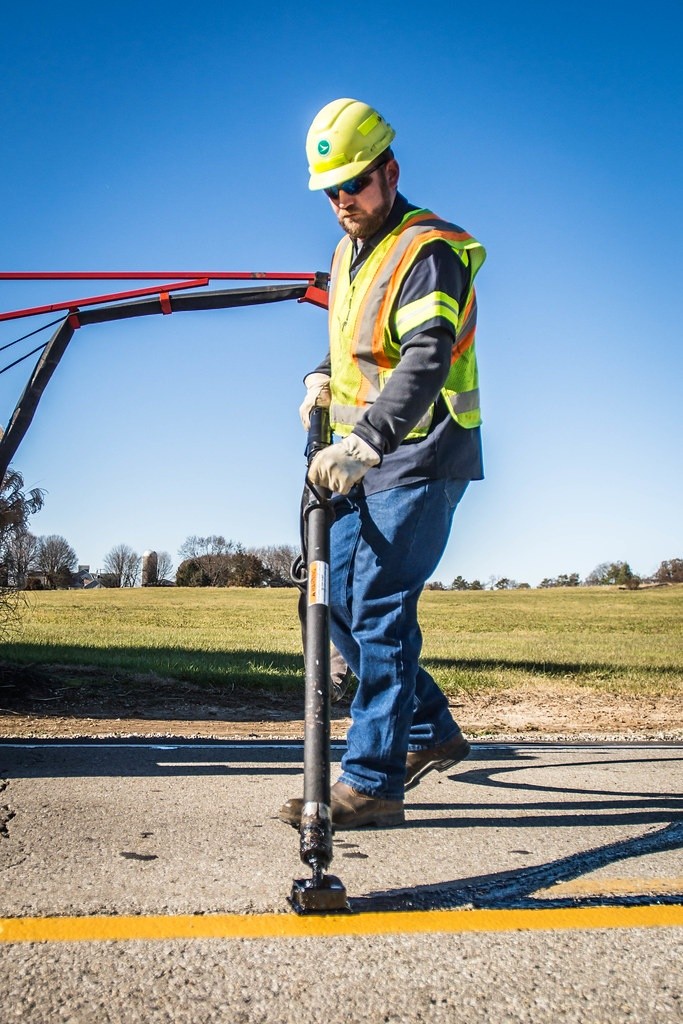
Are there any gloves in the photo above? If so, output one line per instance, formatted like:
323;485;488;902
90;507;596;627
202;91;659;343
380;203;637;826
298;373;330;430
308;434;380;495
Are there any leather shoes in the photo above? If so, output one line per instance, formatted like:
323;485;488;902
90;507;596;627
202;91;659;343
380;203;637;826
403;727;470;790
278;775;406;831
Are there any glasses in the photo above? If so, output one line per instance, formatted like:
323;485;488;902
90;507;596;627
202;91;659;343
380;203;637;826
319;159;393;198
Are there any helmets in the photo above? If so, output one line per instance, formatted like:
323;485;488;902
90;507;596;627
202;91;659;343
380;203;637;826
304;97;398;192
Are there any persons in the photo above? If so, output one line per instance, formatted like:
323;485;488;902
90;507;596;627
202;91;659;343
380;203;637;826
278;97;487;831
